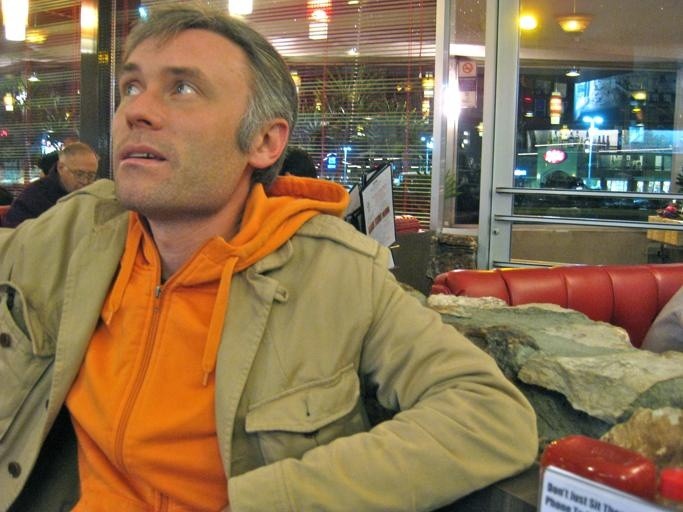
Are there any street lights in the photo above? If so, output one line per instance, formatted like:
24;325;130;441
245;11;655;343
582;116;604;186
420;136;433;172
341;147;351;176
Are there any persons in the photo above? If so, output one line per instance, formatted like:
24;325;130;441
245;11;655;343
6;136;97;227
273;145;316;182
0;7;540;510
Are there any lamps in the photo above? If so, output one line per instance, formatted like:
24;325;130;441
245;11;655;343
555;0;595;37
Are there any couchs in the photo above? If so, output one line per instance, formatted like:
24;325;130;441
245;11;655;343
429;261;683;352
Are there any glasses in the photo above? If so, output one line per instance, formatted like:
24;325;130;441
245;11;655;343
64;164;96;181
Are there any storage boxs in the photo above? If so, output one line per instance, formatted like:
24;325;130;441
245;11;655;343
647;216;683;247
